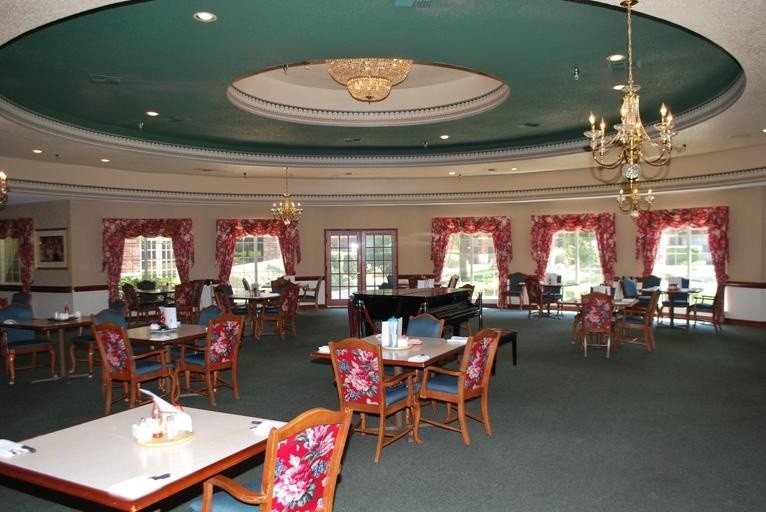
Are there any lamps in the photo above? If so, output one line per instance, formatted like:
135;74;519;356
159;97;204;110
269;166;304;228
324;58;414;104
584;0;687;218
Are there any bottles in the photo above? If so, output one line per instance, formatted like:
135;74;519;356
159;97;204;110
52;304;80;322
382;315;409;348
133;398;186;441
408;273;429;289
149;314;181;332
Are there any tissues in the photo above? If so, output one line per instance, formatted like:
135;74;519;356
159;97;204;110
138;388;193;434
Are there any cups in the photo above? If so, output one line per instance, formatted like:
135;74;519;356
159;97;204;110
433;281;441;288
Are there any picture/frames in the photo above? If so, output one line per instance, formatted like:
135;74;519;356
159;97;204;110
33;228;68;270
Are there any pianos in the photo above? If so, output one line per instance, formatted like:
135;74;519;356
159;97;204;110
352;288;480;337
